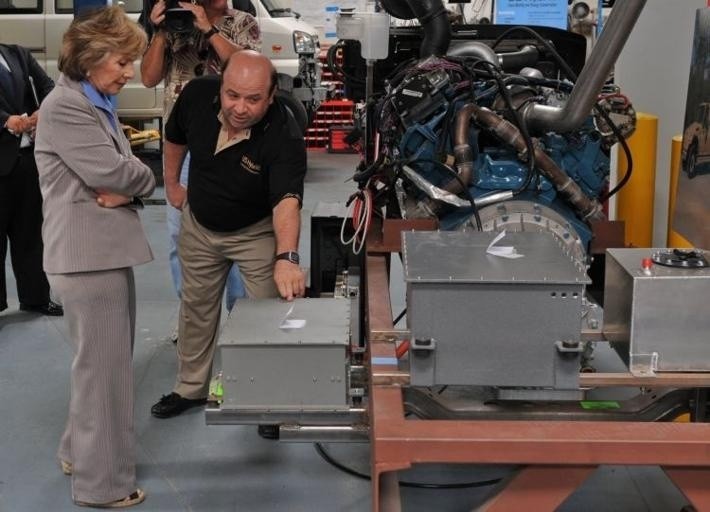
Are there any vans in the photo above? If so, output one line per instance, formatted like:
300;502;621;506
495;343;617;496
1;1;325;133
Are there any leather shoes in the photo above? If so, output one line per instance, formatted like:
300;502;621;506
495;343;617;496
18;300;65;316
150;393;210;419
257;423;280;440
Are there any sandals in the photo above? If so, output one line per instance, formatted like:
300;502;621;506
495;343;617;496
74;487;146;509
61;458;73;475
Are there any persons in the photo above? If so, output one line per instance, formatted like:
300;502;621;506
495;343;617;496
136;0;262;342
145;44;309;441
31;1;156;512
0;43;65;316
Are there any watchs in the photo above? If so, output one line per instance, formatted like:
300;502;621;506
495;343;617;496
272;252;301;264
202;21;221;40
